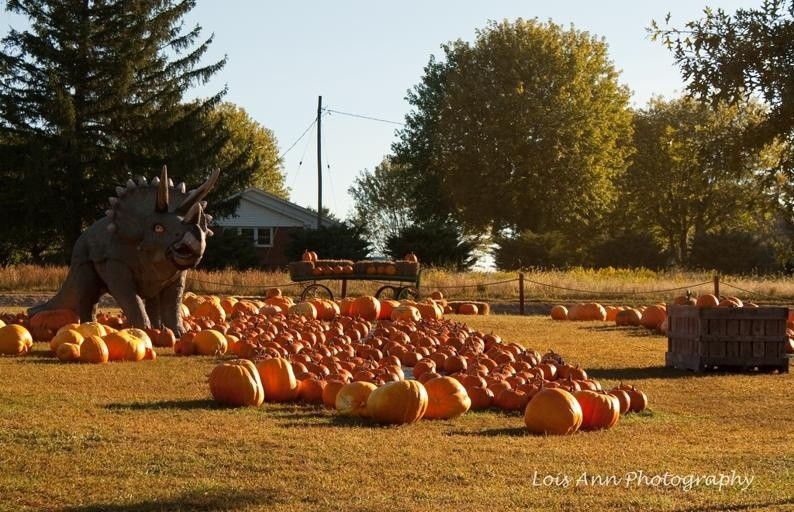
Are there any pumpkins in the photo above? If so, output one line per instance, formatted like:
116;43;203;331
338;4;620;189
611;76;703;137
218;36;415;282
1;288;794;433
301;251;418;274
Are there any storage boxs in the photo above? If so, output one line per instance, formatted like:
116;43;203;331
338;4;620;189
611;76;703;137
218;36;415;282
665;304;789;376
